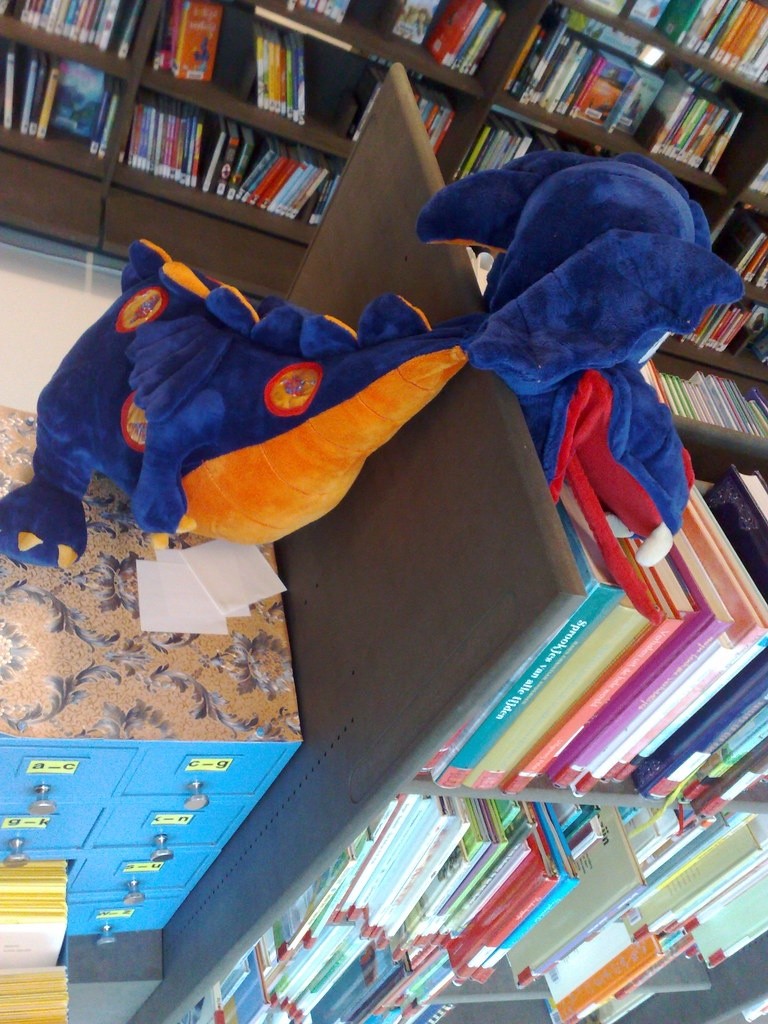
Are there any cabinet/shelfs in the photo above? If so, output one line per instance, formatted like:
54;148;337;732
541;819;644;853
0;0;768;1024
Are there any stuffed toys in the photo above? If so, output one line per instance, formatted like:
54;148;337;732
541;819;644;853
0;152;746;568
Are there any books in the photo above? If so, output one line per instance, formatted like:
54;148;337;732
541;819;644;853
0;0;768;1024
